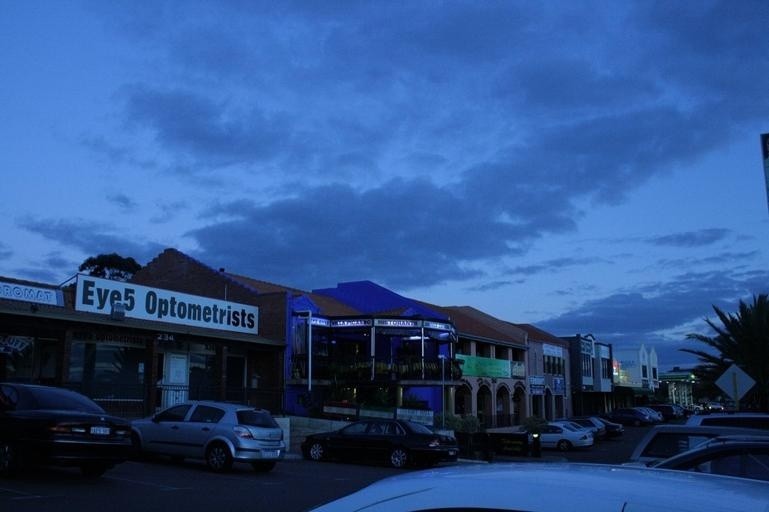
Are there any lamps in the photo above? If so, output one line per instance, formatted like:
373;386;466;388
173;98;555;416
111;302;125;321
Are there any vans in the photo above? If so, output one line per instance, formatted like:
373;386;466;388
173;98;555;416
630;424;769;467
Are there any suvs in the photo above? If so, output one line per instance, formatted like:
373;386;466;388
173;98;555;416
686;412;769;451
301;462;769;511
709;402;724;413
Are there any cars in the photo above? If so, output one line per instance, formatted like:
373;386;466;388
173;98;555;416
650;434;769;481
525;415;626;451
300;417;461;469
614;404;685;426
0;384;131;475
133;400;286;473
693;403;704;411
